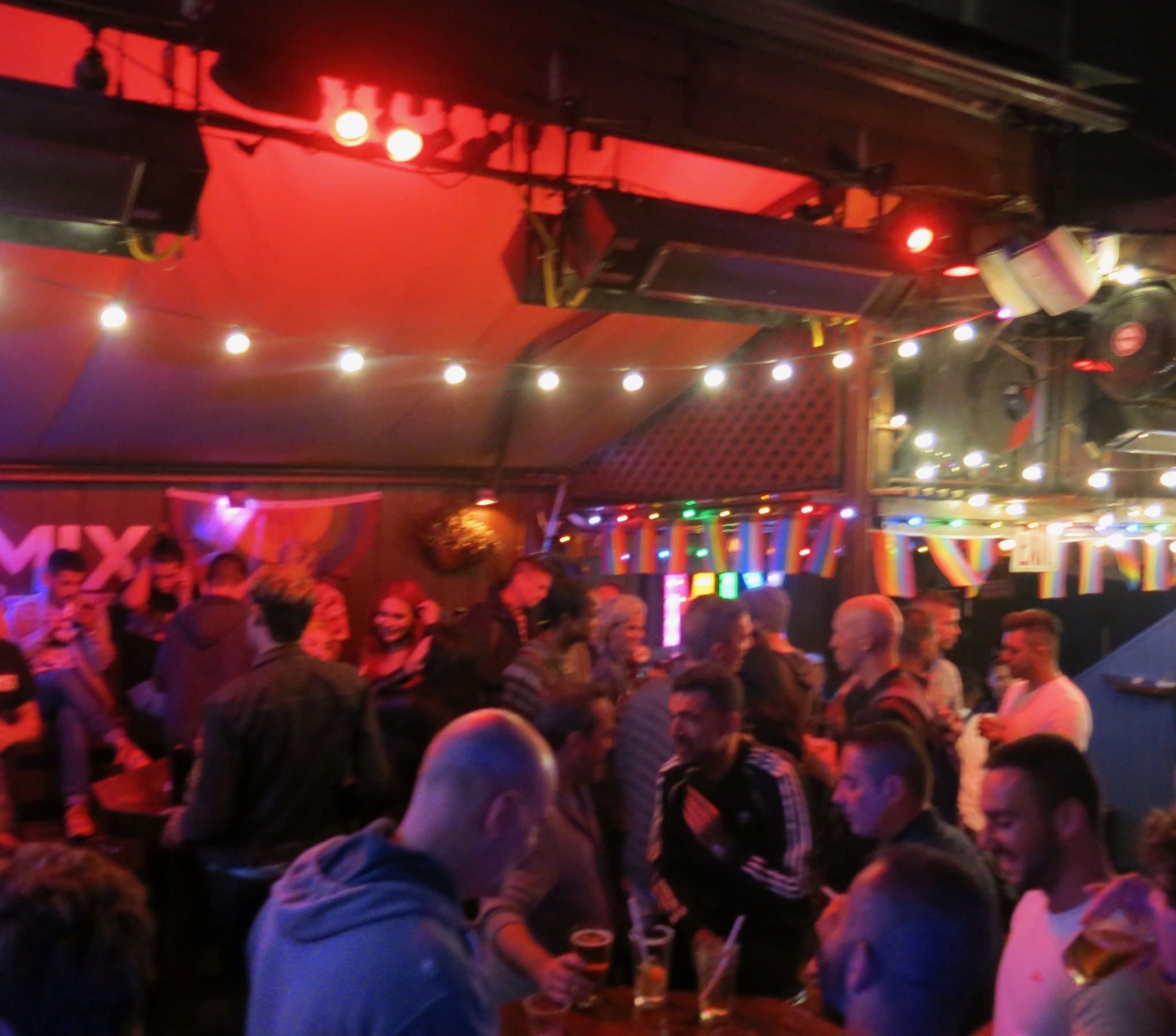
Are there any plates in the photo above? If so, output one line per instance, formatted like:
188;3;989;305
1101;672;1176;694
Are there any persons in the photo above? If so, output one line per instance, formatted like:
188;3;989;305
0;536;1176;1036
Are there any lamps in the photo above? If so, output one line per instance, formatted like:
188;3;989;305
73;25;598;168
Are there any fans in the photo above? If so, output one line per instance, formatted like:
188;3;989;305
1081;279;1176;408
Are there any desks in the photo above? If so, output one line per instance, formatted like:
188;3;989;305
501;990;847;1036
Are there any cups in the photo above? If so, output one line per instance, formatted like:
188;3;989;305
522;992;570;1036
694;941;740;1027
629;925;674;1009
1061;876;1159;987
570;930;614;1008
